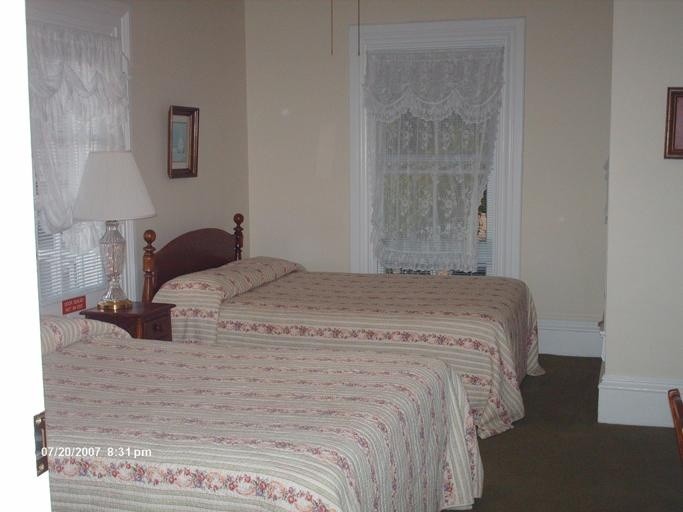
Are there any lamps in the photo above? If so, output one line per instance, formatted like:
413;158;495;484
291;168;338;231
72;149;158;311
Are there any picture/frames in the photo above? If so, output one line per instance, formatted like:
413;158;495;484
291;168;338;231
664;86;683;160
167;104;201;180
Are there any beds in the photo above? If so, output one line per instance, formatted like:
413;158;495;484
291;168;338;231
37;312;485;512
141;213;545;442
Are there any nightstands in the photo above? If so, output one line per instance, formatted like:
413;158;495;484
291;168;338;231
79;301;177;342
